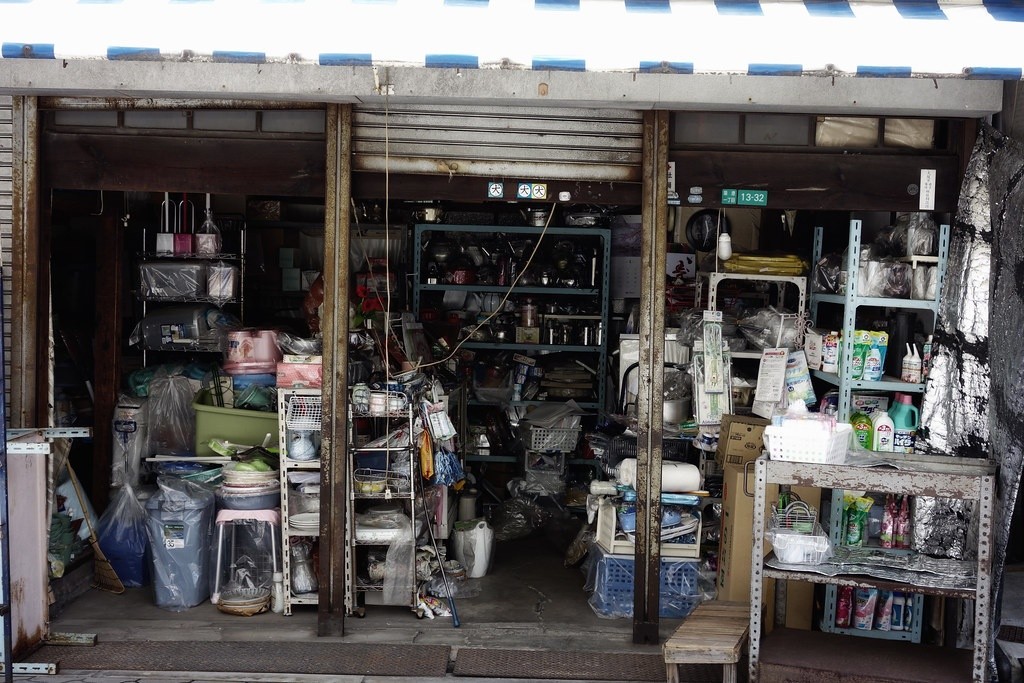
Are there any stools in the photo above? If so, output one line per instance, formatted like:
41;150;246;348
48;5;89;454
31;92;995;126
211;508;284;606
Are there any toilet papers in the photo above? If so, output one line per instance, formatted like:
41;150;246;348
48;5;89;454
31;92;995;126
619;457;701;493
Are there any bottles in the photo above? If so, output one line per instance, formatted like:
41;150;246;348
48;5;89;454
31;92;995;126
778;484;791;528
825;392;919;453
522;293;539;328
902;343;921;382
195;209;223;253
497;256;517;287
427;265;437;284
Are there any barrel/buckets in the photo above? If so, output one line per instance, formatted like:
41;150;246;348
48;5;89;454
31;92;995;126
146;478;215;610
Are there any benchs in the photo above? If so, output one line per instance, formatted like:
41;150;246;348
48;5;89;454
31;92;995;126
662;599;765;683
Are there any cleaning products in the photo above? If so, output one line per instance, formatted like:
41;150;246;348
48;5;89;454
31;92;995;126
819;329;889;382
826;393;919;454
835;583;914;633
839;490;912;557
901;335;933;384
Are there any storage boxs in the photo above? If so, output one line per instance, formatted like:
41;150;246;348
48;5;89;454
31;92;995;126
713;414;770;470
588;543;702;619
195;388;279;456
716;464;822;631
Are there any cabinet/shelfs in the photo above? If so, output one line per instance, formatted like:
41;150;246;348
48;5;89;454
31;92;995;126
695;273;808;414
747;457;1003;683
277;386;323;615
139;225;251;374
344;403;416;614
805;219;950;450
414;221;611;513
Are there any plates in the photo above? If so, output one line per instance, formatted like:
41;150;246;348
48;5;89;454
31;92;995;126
289;512;320;530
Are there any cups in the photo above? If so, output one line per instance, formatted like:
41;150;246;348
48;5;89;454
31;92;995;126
545;322;601;345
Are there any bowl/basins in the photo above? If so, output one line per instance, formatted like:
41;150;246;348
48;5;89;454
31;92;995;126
215;462;280;509
355;481;387;493
